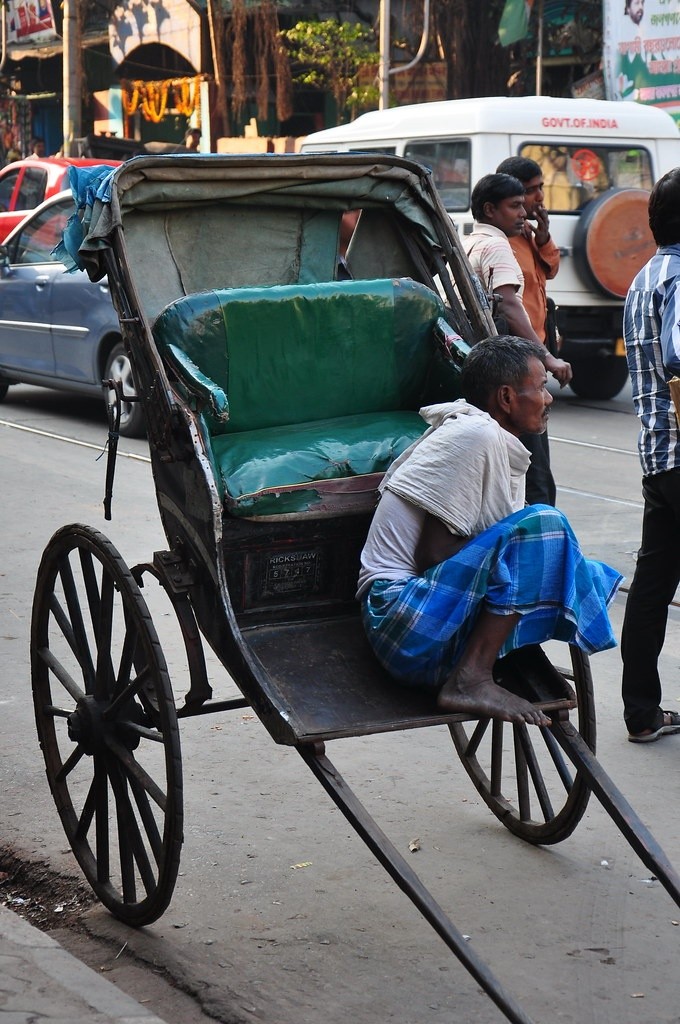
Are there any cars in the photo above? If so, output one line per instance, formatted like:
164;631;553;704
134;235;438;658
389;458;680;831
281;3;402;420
0;188;147;441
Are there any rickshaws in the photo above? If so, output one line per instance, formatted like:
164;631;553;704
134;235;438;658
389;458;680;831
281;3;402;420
28;151;680;1024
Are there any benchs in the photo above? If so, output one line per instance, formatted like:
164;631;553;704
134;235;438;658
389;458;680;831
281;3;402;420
150;276;475;518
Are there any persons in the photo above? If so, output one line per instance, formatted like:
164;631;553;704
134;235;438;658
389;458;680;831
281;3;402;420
356;337;628;726
623;0;644;41
443;156;572;507
22;136;46;160
621;167;680;743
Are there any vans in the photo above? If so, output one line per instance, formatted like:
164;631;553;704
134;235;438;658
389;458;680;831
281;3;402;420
59;131;150;162
295;96;679;400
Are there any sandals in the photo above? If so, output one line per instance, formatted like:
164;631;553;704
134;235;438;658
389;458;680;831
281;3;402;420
628;711;680;743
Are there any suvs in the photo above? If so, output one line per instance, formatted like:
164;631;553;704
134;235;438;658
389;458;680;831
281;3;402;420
0;152;126;249
